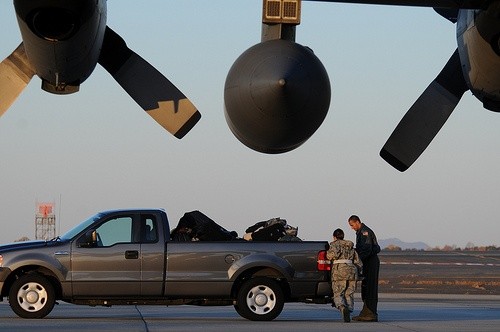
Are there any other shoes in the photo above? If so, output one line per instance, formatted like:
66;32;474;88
342;306;350;322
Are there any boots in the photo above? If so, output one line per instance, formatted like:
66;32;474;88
352;304;378;321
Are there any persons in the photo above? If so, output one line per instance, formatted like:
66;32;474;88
348;215;381;321
326;228;364;322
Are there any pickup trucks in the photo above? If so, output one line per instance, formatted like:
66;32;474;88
0;207;334;322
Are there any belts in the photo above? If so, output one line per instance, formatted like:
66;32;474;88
333;260;352;263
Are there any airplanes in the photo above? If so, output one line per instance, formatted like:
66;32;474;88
0;0;500;175
